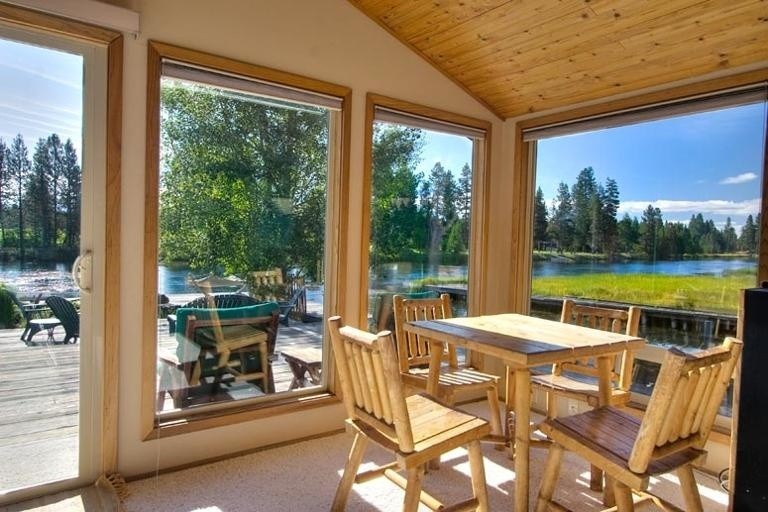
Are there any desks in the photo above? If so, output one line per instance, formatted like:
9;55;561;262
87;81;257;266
401;310;646;511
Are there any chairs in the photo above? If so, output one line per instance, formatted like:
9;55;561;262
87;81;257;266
156;292;325;412
325;314;491;511
390;292;507;472
45;294;80;344
535;337;747;510
501;299;647;492
3;288;61;345
